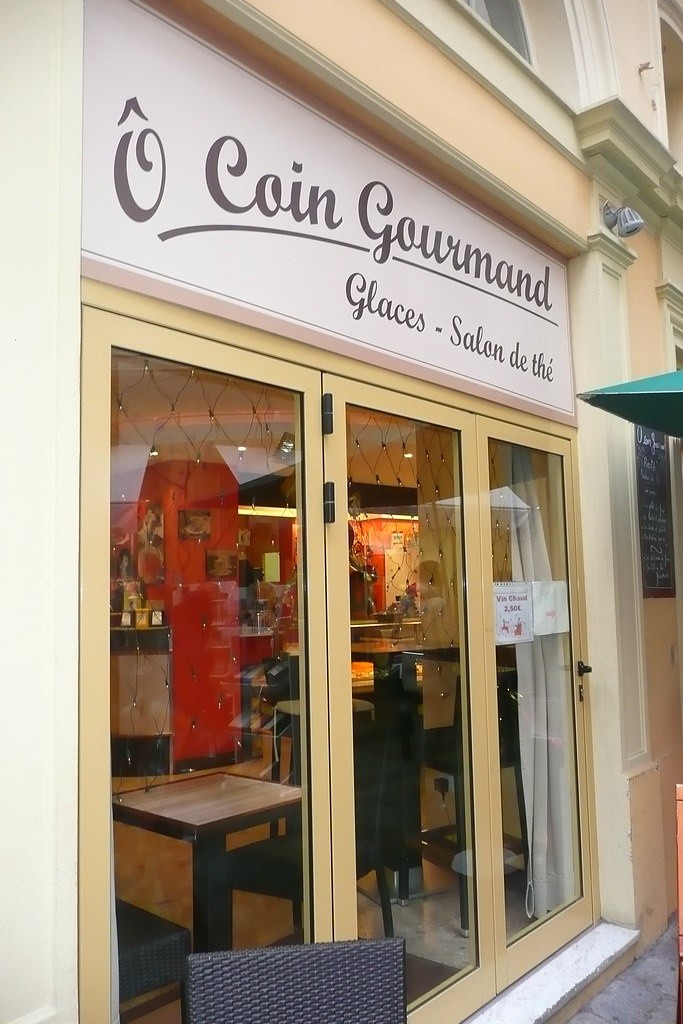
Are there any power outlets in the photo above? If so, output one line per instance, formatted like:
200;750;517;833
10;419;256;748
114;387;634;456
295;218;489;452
438;772;455;793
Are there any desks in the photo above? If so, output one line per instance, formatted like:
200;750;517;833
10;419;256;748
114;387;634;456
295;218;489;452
115;770;303;947
348;642;517;905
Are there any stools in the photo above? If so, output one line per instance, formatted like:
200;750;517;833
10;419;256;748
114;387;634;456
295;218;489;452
270;697;376;840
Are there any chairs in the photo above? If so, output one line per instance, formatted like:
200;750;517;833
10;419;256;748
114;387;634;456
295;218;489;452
391;668;529;937
218;718;395;938
180;935;408;1024
115;897;192;1024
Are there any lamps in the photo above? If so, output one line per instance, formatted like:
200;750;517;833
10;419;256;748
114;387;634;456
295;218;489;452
603;205;646;238
